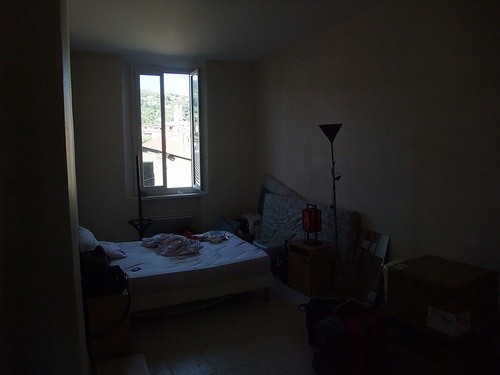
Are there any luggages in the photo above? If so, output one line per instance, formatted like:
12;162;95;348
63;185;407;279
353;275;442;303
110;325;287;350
343;307;386;375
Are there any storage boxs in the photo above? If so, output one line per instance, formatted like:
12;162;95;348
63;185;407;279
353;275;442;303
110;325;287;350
383;253;500;340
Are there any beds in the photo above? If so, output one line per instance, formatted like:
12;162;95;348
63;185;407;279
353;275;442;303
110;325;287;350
78;222;275;312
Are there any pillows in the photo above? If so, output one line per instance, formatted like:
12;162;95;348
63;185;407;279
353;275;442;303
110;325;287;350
78;225;127;258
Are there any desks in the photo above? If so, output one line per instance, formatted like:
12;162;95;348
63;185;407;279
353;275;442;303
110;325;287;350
286;241;329;292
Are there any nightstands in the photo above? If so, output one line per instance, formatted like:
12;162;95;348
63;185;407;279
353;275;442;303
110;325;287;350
85;294;136;358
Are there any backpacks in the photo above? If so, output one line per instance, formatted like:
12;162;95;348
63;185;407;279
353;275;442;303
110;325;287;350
310;318;349;375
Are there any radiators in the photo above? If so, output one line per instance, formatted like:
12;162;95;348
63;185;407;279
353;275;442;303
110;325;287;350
142;213;195;239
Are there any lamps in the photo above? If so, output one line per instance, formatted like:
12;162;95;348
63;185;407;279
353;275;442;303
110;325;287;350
316;124;343;254
301;201;324;246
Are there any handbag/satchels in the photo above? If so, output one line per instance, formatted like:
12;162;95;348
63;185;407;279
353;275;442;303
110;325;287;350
296;297;341;347
82;265;129;297
